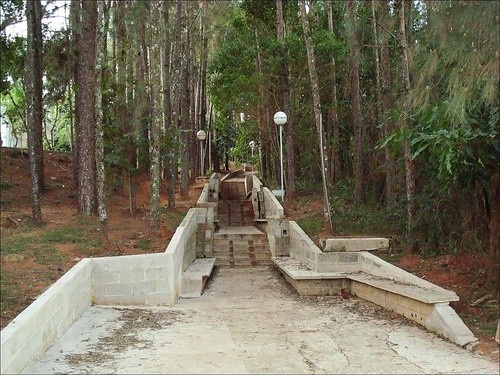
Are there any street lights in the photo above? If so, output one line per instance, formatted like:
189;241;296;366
197;130;207;177
274;111;287;201
